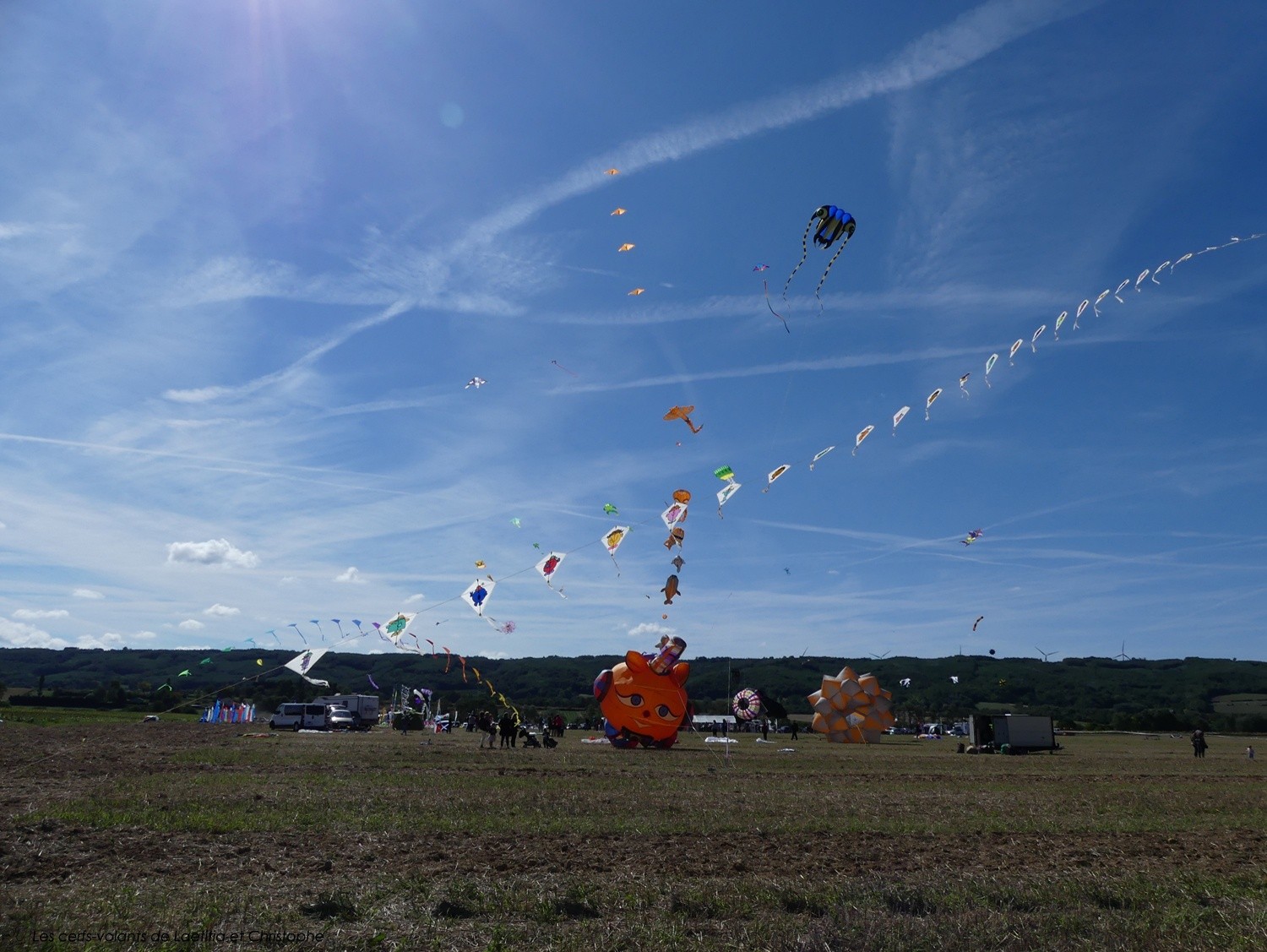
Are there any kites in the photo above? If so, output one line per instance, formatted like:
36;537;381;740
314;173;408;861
511;706;521;727
499;693;507;708
664;404;704;449
600;487;690;620
464;359;583;394
783;204;857;313
713;233;1263;634
753;262;791;335
531;541;570;602
605;167;644;297
497;621;516;635
158;559;497;698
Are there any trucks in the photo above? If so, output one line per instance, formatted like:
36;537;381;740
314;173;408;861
312;695;379;731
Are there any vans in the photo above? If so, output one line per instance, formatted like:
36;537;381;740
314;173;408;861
269;703;326;732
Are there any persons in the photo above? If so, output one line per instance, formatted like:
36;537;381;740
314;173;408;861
377;704;923;749
1191;729;1255;760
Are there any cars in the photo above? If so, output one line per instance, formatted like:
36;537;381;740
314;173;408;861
327;710;354;729
1054;728;1062;735
890;728;902;735
775;726;791;734
143;716;158;723
566;724;577;729
